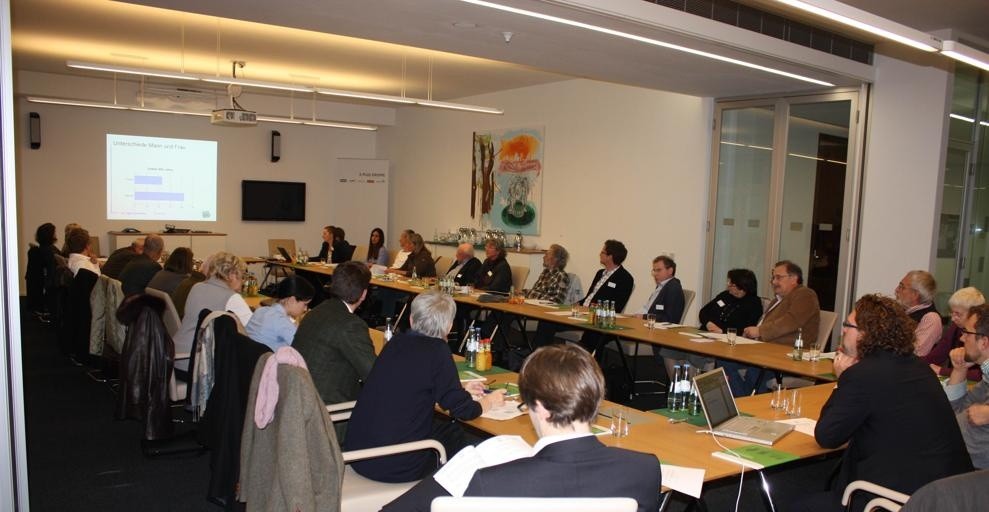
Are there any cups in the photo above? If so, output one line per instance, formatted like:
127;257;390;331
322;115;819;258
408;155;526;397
647;313;656;330
726;328;736;349
423;277;431;288
613;407;628;439
571;297;579;316
467;283;474;293
786;390;801;417
770;384;787;410
809;344;821;361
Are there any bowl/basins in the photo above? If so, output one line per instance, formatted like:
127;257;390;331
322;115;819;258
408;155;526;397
523;243;538;249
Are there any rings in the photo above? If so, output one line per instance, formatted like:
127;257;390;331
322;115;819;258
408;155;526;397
836;356;842;361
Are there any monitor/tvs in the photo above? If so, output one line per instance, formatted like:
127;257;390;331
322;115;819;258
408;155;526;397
241;179;307;222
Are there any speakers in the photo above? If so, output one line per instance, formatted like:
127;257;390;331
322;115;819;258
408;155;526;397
271;130;281;161
30;112;42;149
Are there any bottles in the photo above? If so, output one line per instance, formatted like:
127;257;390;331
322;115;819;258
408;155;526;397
433;228;455;242
688;367;703;415
793;328;804;360
293;248;310;266
680;364;690;410
411;266;418;282
383;318;395;347
456;227;524;251
239;271;262;296
588;300;619;330
466;326;493;371
667;365;679;412
434;272;459;297
508;286;530;305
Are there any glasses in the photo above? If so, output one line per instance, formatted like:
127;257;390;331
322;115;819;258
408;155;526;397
842;320;858;328
517;402;529;412
960;329;984;337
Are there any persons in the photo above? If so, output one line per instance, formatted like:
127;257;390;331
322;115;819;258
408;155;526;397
307;225;513;350
36;223;253;400
476;243;571;372
715;260;821;400
916;285;987;383
287;259;381;445
344;288;507;484
461;340;662;511
659;268;763;389
940;302;989;470
533;238;635;360
239;274;319;356
892;267;944;359
575;254;686;361
813;293;973;512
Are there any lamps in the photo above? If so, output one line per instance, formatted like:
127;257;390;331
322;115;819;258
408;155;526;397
270;129;282;164
28;112;42;150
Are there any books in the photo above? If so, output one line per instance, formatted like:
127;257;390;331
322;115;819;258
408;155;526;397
710;443;801;471
483;381;521;398
784;348;839;363
456;370;488;383
590;423;612;437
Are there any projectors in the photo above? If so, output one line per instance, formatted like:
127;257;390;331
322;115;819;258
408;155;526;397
211;109;258;127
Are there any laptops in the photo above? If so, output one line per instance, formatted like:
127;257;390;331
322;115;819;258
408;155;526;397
692;367;796;447
278;245;305;264
